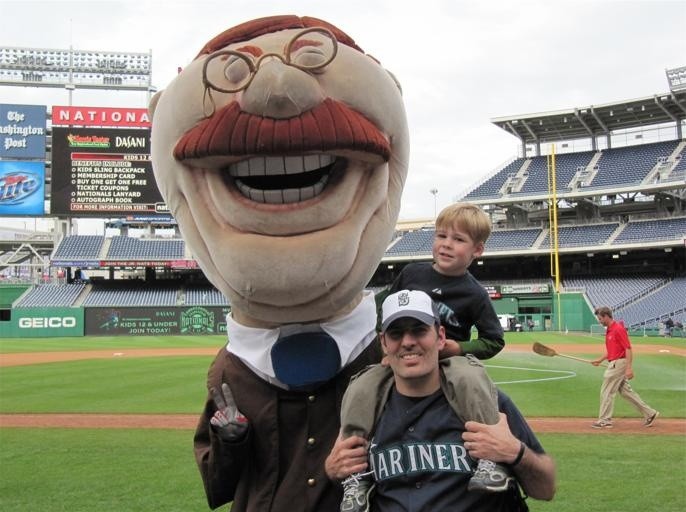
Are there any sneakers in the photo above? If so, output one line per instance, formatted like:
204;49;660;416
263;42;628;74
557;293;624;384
643;410;660;427
339;468;377;511
590;421;613;429
468;456;516;495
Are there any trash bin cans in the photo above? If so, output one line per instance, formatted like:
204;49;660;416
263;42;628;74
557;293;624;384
515;324;522;332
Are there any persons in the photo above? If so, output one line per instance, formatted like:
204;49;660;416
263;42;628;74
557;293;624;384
146;14;410;512
325;290;556;512
587;306;660;430
340;202;515;512
619;317;624;329
658;317;684;339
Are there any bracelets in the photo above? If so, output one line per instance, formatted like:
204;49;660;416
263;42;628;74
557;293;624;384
512;443;525;467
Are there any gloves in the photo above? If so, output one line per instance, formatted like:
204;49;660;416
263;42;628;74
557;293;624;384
209;381;249;440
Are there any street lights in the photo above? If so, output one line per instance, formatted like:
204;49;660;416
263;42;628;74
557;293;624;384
430;188;438;220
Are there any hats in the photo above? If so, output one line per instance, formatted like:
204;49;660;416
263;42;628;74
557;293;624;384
380;288;442;336
